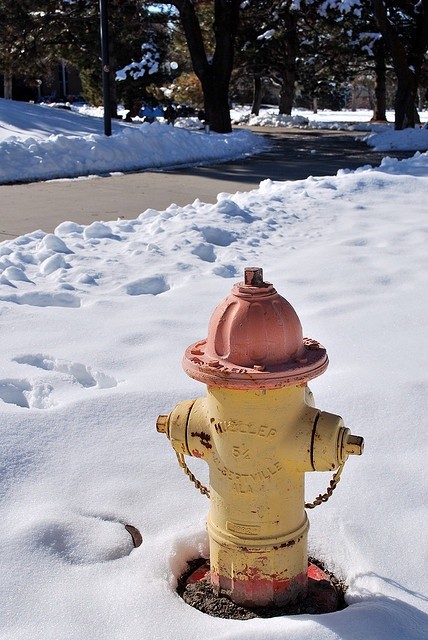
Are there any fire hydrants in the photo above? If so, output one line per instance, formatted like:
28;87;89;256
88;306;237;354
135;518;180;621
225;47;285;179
154;266;366;622
